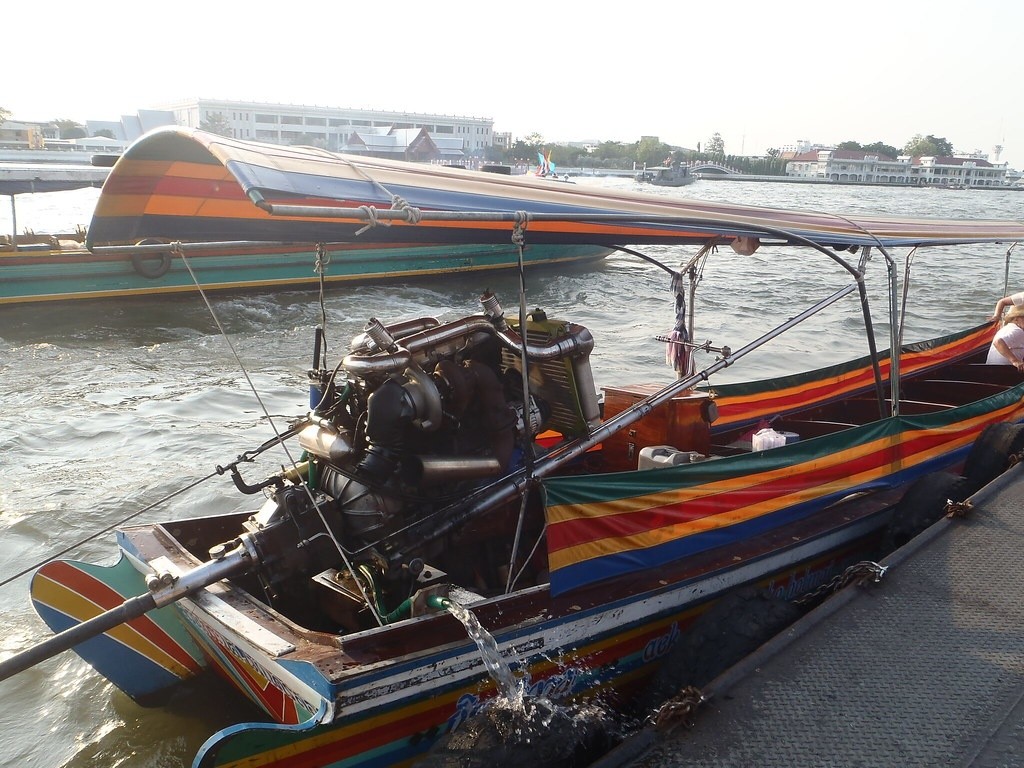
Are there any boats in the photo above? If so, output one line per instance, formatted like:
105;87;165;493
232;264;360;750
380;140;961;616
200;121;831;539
1;163;628;305
651;165;698;187
27;126;1024;768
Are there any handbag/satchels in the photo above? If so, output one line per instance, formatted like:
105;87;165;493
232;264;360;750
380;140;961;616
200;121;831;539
752;429;786;453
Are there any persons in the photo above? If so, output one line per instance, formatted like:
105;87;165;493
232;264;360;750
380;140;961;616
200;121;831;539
984;290;1024;372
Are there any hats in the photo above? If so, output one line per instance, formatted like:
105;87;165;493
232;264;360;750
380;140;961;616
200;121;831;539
1007;306;1024;318
